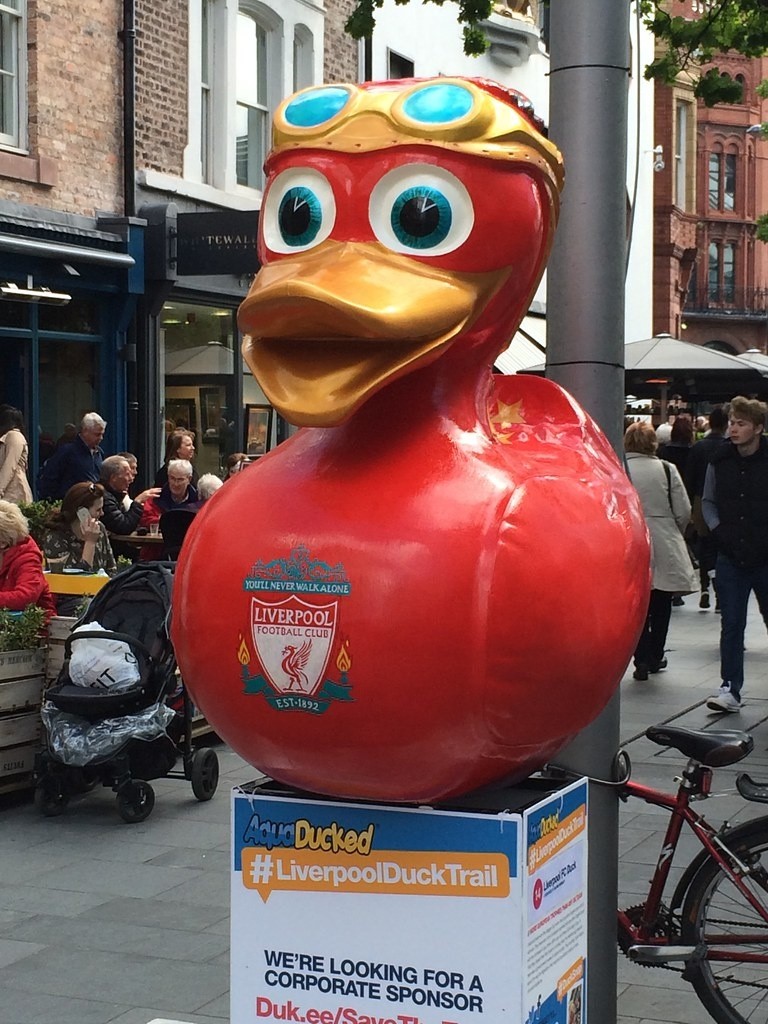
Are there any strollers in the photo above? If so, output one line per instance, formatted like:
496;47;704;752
33;560;219;824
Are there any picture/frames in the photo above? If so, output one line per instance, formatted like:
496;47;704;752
244;403;274;460
165;397;197;455
198;388;221;445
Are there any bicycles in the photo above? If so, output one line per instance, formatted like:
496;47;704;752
617;724;768;1024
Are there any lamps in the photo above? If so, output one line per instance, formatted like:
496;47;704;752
643;145;665;172
0;282;72;306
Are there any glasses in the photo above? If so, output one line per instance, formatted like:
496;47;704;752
89;482;95;493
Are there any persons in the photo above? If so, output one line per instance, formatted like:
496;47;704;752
44;480;118;577
188;472;223;513
0;404;34;509
155;428;197;485
0;499;56;636
702;397;768;713
154;458;198;511
95;455;163;560
618;420;701;681
38;411;108;504
654;402;730;613
224;453;250;480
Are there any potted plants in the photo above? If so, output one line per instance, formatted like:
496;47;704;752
0;603;49;808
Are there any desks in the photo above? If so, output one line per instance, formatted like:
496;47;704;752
112;532;165;559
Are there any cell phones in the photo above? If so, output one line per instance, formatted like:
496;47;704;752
77;507;93;526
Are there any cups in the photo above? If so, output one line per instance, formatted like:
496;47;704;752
150;524;159;536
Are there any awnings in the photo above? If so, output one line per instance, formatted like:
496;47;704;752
493;312;761;376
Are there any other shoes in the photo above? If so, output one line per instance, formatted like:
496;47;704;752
673;597;684;605
707;681;742;712
715;599;722;612
700;594;711;608
648;658;667;673
633;670;648;680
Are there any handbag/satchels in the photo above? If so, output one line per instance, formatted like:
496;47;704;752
39;468;77;498
68;620;141;689
